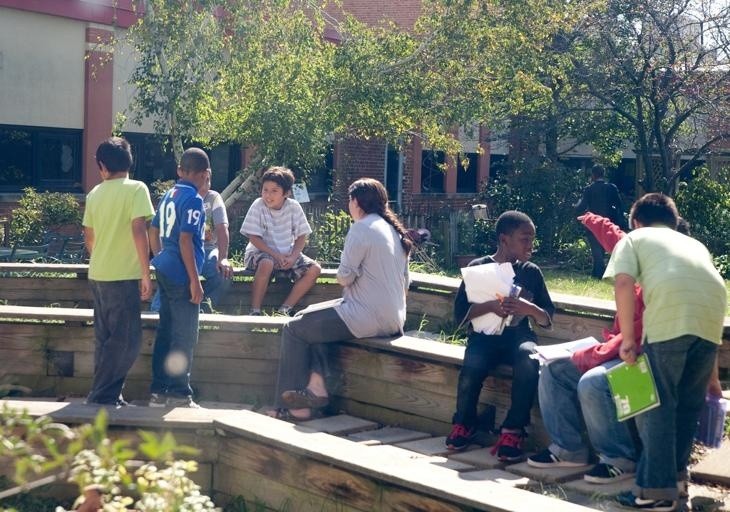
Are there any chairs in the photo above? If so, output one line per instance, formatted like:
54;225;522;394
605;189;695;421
7;244;50;263
56;236;87;264
0;218;11;248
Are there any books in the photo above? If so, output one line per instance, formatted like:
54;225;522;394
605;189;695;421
604;351;659;421
534;335;602;361
504;284;524;329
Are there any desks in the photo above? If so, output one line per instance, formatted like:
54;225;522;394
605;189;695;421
0;248;40;263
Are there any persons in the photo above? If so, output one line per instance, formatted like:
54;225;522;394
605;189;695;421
239;164;322;317
576;160;628;277
523;209;691;484
265;175;414;422
195;166;237;312
443;210;555;462
149;148;209;408
81;137;155;405
601;194;727;511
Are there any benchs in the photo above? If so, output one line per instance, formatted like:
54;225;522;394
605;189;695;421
0;263;730;491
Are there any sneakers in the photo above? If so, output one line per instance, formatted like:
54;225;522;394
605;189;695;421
584;462;634;484
445;415;475;450
618;491;675;511
249;309;262;316
272;304;296;317
268;388;329;421
497;429;525;462
527;448;590;468
149;394;201;408
